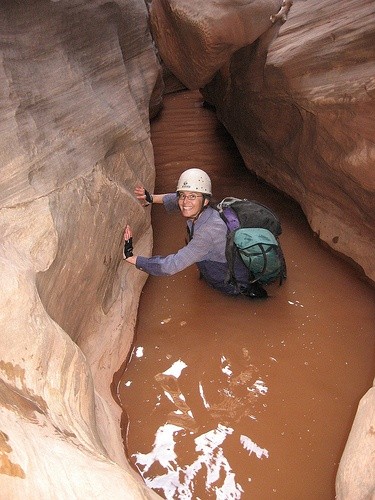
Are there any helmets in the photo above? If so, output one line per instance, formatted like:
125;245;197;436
176;168;212;195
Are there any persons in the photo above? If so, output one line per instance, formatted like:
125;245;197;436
123;164;268;296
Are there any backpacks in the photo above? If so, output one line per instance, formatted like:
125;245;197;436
227;226;287;288
216;196;284;238
